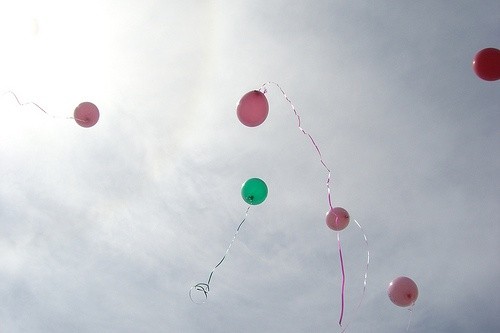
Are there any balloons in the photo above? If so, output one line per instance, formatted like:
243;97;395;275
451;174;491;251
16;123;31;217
241;178;269;205
74;102;100;128
236;90;269;127
472;47;500;81
325;207;350;232
388;277;419;307
